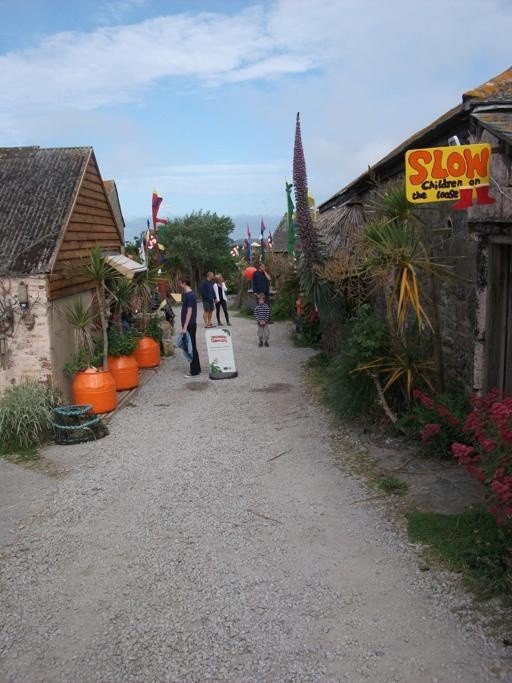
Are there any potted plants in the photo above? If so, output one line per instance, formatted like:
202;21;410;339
63;241;164;414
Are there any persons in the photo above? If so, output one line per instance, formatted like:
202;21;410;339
200;271;232;328
177;276;201;378
253;261;274;348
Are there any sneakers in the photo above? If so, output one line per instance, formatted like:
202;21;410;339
218;323;231;326
259;341;269;347
184;372;202;378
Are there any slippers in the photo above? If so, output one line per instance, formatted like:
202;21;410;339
205;323;216;328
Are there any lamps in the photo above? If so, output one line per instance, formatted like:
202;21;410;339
17;281;29;310
